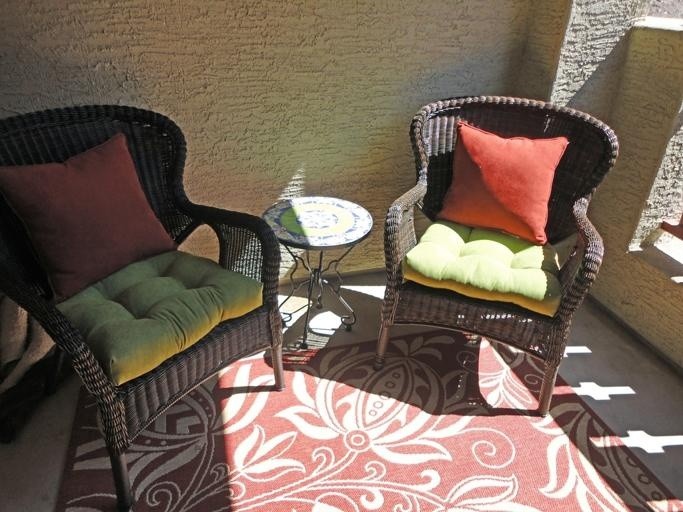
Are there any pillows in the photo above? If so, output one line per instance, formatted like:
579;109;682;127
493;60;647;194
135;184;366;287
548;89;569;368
0;133;179;303
436;120;570;245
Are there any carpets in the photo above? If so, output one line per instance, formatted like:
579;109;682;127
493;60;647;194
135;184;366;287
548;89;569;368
54;318;682;512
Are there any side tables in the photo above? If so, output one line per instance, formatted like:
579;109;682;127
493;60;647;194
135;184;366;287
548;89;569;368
262;196;374;348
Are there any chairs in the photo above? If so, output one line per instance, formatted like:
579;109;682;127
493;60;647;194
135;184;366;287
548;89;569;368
0;104;286;512
373;94;619;417
